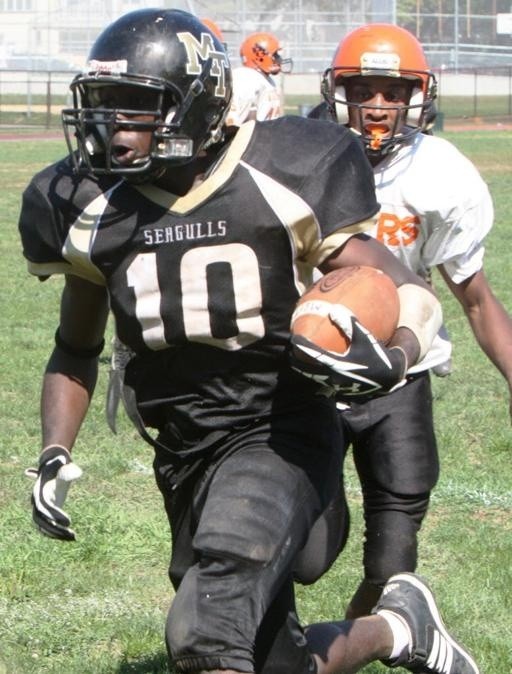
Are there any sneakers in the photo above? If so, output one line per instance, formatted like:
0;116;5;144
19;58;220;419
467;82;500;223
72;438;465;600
367;571;480;673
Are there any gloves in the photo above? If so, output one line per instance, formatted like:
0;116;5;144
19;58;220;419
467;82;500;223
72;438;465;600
286;308;411;406
24;442;84;544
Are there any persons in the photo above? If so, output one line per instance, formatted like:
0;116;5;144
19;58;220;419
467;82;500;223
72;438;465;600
290;24;511;628
224;30;294;121
12;5;479;672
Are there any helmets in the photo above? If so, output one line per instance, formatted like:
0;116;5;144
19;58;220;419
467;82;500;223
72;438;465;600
69;7;234;173
238;31;283;75
326;23;432;132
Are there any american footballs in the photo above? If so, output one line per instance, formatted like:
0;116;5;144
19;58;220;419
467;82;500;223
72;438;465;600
289;265;401;367
